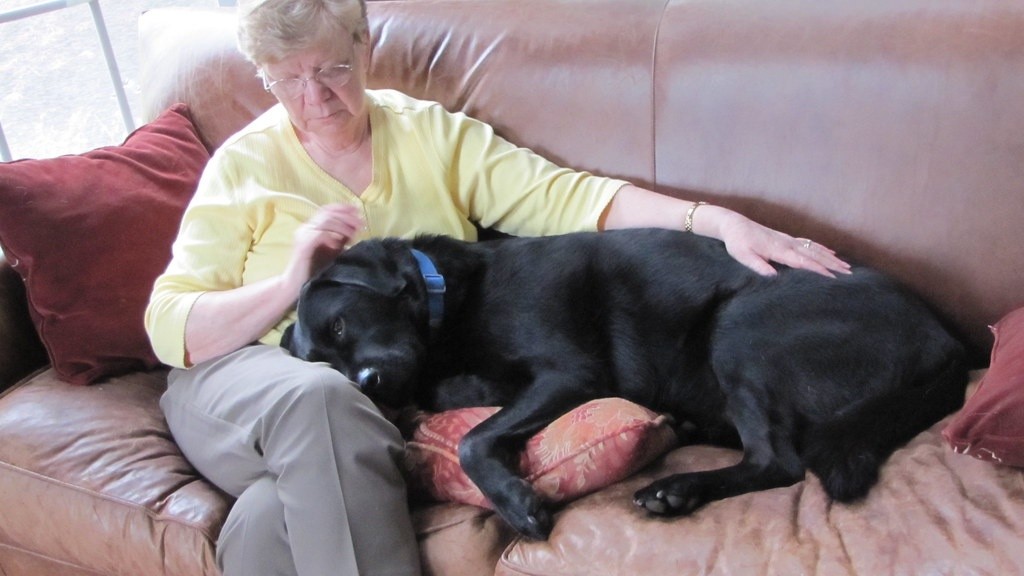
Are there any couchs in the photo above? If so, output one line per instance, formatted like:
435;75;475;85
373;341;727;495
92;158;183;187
0;0;1024;576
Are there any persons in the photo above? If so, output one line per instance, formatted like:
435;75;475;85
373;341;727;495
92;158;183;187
143;0;852;576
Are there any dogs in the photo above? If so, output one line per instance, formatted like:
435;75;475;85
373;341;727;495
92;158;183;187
279;227;994;545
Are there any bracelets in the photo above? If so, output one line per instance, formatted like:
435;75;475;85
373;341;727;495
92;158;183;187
684;201;709;234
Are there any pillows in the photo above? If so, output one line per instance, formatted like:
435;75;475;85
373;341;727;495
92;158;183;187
396;398;678;509
0;102;210;386
941;307;1024;466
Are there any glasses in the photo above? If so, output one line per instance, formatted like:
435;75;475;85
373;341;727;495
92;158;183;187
262;32;356;102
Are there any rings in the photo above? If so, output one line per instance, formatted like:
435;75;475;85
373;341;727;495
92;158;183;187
803;239;812;248
